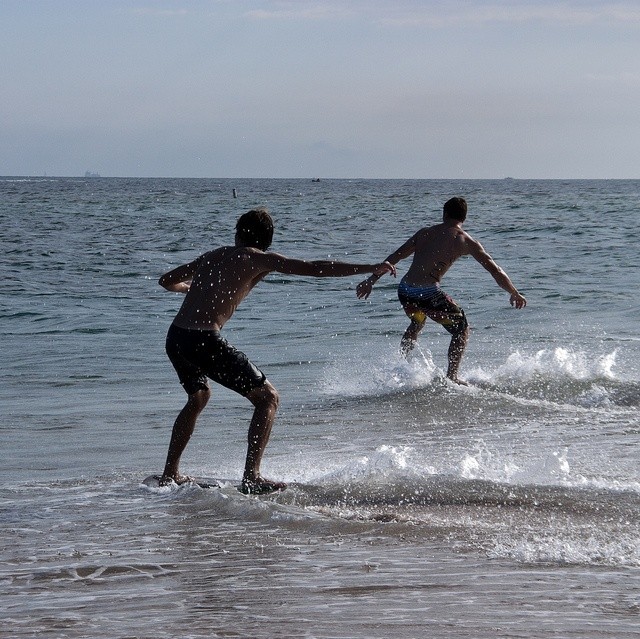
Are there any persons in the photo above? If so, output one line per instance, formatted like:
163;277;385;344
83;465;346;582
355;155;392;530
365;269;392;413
356;197;527;386
158;208;397;494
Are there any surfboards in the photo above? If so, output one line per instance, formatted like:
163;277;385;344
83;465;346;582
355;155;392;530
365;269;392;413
143;469;345;509
